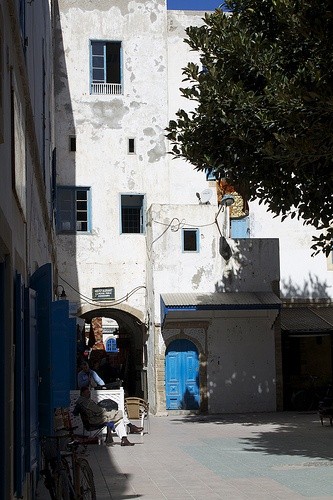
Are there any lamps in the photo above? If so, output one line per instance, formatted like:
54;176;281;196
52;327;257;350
55;285;66;301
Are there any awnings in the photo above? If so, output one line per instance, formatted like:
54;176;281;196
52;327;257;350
280;307;333;335
160;291;282;333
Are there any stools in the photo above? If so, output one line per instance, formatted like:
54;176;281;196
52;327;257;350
48;431;69;470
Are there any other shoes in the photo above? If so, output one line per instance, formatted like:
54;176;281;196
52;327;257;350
130;425;143;434
121;439;135;446
106;437;113;443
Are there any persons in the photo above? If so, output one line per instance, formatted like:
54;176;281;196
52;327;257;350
78;360;107;390
77;386;144;446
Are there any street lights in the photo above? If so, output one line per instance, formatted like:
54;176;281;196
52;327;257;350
215;195;235;221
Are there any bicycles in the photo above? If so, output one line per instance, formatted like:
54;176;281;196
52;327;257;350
38;426;97;500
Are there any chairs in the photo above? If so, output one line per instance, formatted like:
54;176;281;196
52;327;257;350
74;405;109;449
126;397;150;437
